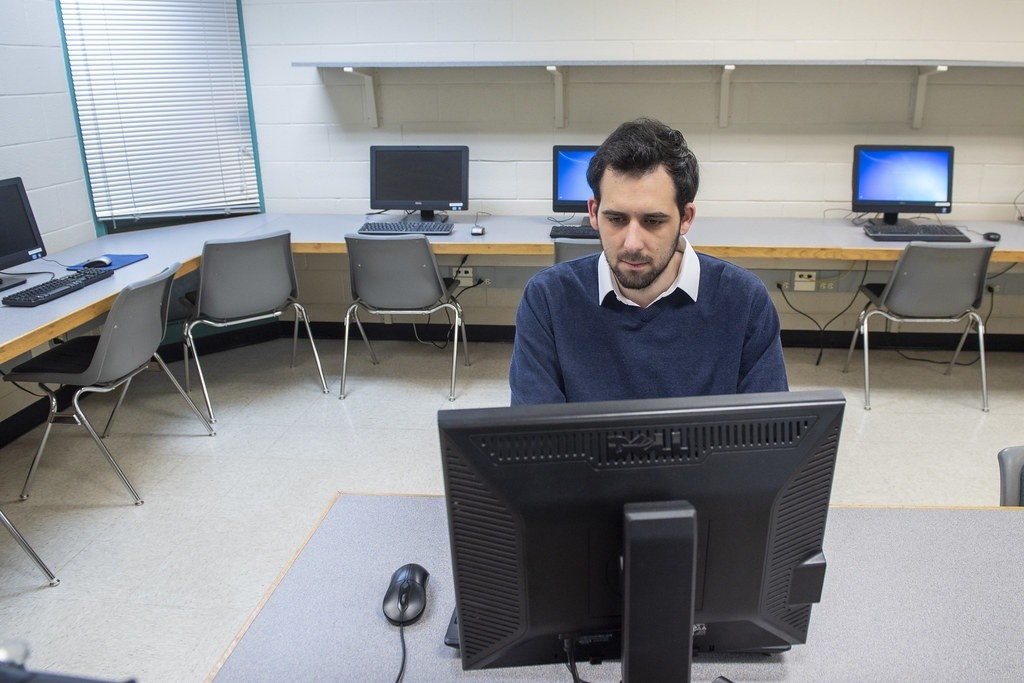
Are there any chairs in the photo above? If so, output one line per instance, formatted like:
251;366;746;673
840;241;995;411
183;230;329;423
3;260;216;506
339;233;470;402
554;241;604;265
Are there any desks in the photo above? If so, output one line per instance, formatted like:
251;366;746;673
209;489;1023;683
0;219;1024;371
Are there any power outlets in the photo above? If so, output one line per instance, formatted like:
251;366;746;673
451;268;476;287
794;271;817;292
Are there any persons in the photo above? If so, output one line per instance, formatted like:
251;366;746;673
510;114;788;407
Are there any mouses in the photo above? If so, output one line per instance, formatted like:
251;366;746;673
471;226;485;236
983;232;1001;242
383;564;430;626
82;256;112;267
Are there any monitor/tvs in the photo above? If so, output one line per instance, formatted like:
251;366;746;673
438;390;846;683
552;145;600;225
0;176;47;293
851;145;954;227
370;146;469;223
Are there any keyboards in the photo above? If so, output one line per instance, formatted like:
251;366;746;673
2;266;115;307
357;222;454;236
864;226;971;242
550;226;601;238
